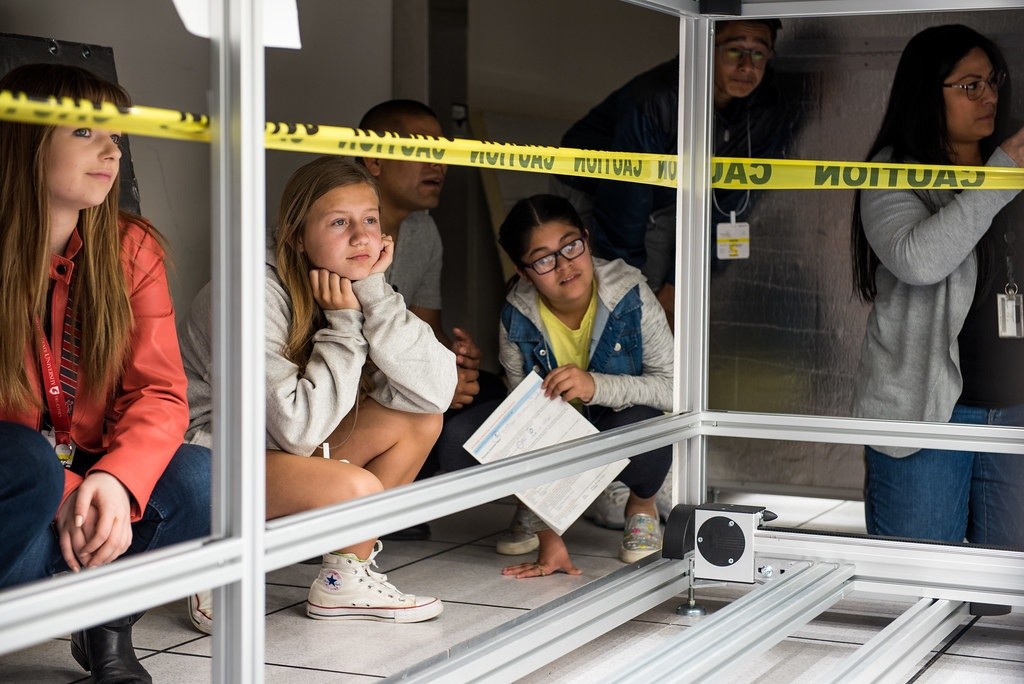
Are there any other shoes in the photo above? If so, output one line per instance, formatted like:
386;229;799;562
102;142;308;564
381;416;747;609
378;519;430;540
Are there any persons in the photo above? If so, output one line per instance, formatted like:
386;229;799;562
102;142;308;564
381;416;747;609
559;18;801;312
0;62;213;684
355;99;508;541
495;194;674;579
852;23;1024;547
174;154;459;635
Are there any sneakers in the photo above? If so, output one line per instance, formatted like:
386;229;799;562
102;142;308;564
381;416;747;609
583;478;632;530
188;589;214;636
496;500;540;555
306;541;444;622
619;497;663;564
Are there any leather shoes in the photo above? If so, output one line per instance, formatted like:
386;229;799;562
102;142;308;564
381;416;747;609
71;624;152;684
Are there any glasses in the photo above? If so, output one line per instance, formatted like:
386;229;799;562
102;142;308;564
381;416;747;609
520;234;587;276
714;45;772;68
943;67;1008;101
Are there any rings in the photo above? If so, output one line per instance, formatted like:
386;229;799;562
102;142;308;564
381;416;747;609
537;566;545;576
